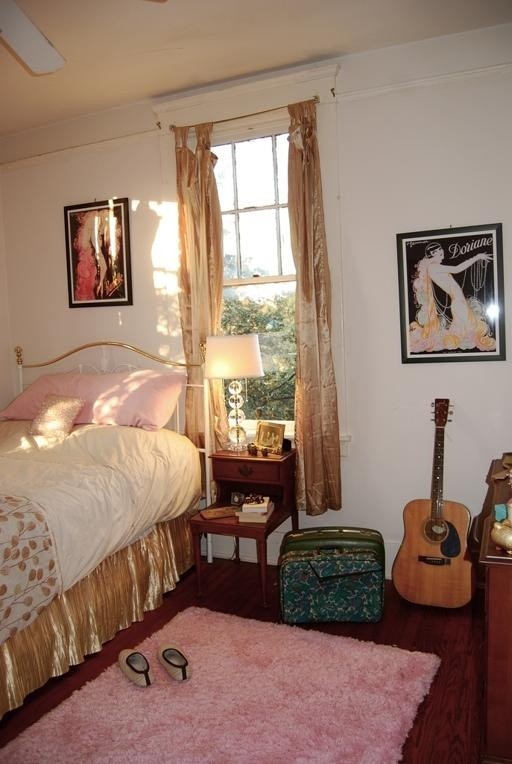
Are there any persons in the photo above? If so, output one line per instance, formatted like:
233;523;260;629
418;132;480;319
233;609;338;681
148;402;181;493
75;209;121;300
259;430;279;449
409;243;496;352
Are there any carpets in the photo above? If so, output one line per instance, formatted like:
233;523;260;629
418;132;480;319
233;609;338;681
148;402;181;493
0;610;443;764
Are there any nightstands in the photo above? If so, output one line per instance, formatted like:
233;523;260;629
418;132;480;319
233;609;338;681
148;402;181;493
187;449;298;607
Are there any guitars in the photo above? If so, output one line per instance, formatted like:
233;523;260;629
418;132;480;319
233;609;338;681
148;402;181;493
391;398;476;609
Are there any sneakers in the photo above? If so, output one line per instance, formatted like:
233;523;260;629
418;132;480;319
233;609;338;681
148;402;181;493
118;651;149;691
160;646;190;684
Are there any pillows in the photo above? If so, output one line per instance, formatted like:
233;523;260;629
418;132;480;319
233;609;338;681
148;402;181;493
0;368;187;431
28;394;85;443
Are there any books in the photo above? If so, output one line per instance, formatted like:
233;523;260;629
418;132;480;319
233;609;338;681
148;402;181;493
241;495;275;526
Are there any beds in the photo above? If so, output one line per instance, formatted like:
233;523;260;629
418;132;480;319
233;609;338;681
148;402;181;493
0;341;217;719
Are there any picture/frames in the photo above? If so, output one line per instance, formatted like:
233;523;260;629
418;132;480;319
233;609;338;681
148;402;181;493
256;422;285;455
63;197;133;308
396;223;506;364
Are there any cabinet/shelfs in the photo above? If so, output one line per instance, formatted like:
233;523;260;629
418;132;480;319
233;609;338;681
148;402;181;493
470;460;512;764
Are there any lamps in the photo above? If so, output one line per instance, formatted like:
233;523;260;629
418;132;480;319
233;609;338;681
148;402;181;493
204;334;265;451
